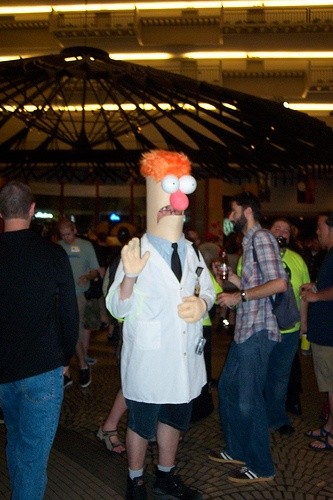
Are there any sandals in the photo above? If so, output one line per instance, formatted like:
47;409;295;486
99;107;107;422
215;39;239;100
96;423;126;455
302;427;333;452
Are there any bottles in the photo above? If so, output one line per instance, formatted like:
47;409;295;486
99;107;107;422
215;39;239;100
218;247;229;280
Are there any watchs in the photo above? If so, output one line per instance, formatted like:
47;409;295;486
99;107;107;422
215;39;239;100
239;288;247;302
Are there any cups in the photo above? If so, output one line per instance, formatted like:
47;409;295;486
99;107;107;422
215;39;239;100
301;332;311;351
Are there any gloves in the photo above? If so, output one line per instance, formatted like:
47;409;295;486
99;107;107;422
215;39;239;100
121;237;150;277
178;294;208;324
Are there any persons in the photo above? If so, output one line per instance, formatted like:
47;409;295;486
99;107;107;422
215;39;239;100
0;178;80;500
28;196;324;391
95;230;224;454
207;191;289;483
236;217;313;438
298;205;333;453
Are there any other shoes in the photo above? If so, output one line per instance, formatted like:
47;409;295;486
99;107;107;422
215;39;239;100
85;357;98;366
64;364;92;388
153;464;202;500
125;474;148;500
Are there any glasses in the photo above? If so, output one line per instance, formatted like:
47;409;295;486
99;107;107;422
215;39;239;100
159;208;182;216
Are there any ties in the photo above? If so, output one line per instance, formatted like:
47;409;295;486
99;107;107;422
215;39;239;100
171;243;182;284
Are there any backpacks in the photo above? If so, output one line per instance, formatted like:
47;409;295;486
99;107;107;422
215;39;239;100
251;229;302;331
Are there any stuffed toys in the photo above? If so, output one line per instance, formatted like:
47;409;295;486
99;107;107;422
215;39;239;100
106;149;217;500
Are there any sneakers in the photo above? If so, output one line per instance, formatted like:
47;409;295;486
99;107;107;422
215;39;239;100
227;465;275;482
207;448;246;465
